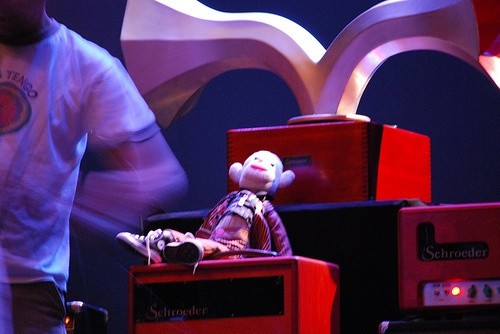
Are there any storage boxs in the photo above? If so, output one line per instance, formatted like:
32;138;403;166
226;113;431;205
128;250;340;334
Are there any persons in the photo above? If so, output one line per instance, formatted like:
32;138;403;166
0;0;189;333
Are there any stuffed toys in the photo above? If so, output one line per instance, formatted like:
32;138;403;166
114;149;299;265
